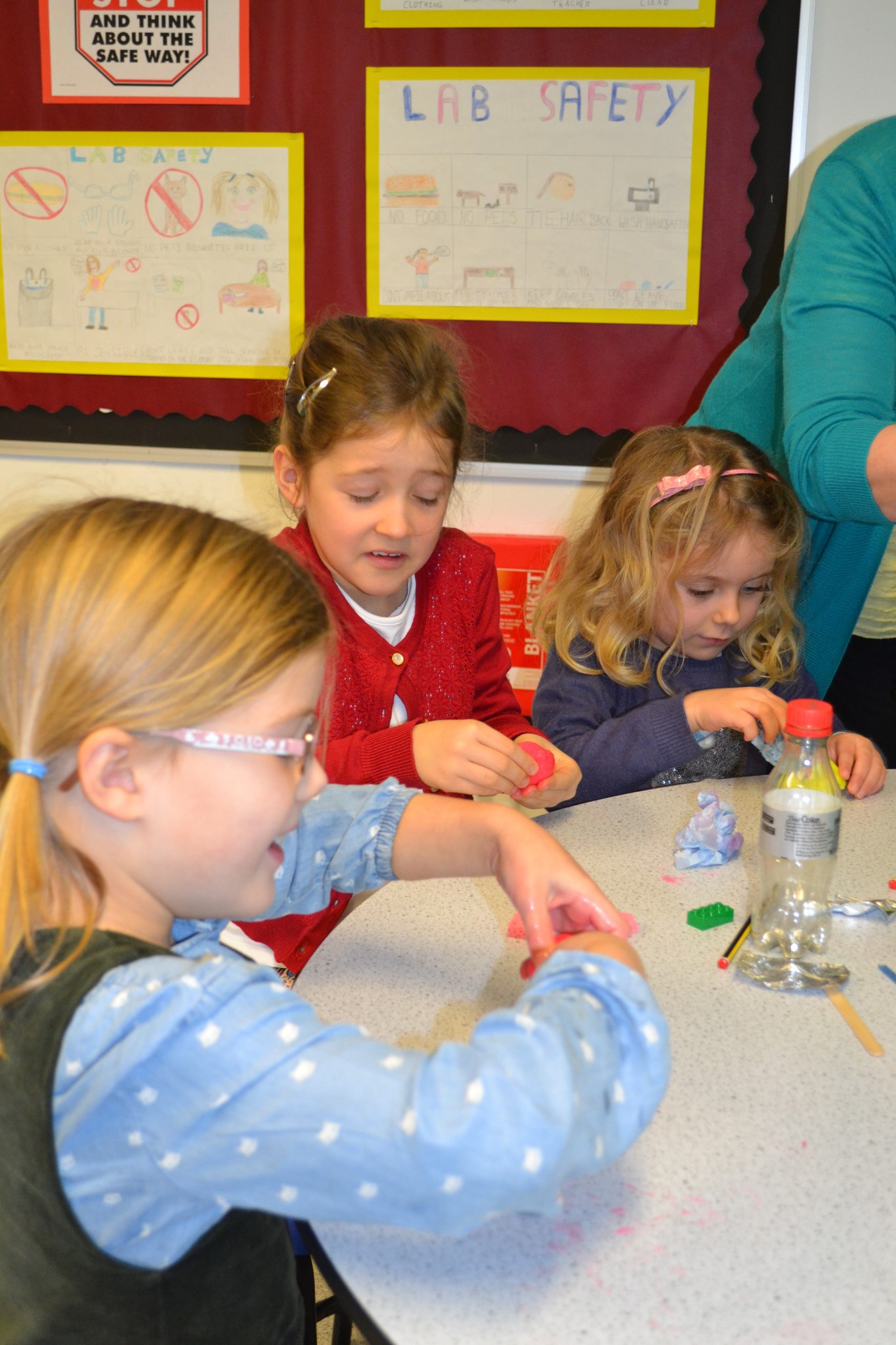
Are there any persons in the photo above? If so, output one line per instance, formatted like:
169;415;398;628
0;495;670;1345
526;425;886;812
677;115;896;769
209;312;582;989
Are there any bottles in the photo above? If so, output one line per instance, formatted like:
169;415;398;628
750;698;843;958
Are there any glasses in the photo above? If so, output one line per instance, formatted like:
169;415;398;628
57;721;318;794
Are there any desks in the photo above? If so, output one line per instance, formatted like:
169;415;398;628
293;775;896;1345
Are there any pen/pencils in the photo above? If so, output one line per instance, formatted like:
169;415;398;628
717;915;751;969
